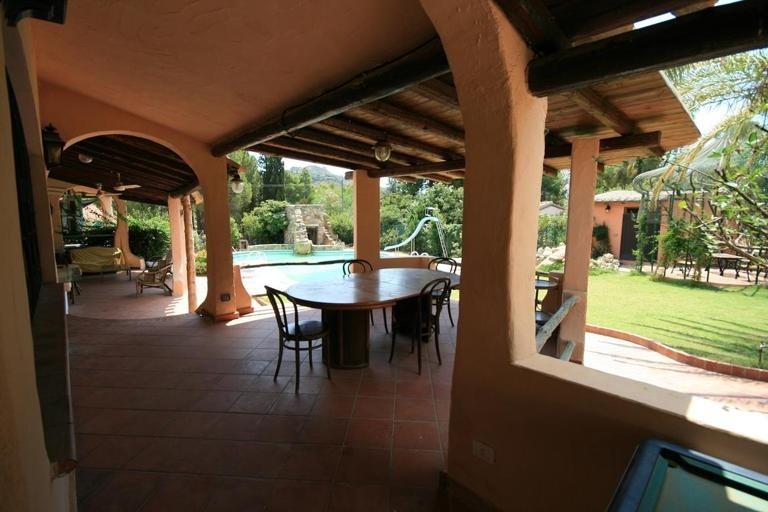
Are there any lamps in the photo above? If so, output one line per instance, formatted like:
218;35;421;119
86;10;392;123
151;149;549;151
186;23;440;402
229;167;246;194
371;130;395;162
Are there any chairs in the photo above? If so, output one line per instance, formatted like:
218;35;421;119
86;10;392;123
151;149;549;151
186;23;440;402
343;255;456;376
135;260;173;296
263;285;332;396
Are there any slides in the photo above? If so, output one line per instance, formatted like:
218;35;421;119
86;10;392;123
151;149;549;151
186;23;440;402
384;217;431;251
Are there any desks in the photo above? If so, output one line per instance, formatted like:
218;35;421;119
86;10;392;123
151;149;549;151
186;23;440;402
285;266;460;370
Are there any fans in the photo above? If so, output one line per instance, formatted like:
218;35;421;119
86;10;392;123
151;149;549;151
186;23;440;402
85;173;141;199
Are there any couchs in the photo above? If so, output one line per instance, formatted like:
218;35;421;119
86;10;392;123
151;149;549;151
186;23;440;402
70;245;131;282
